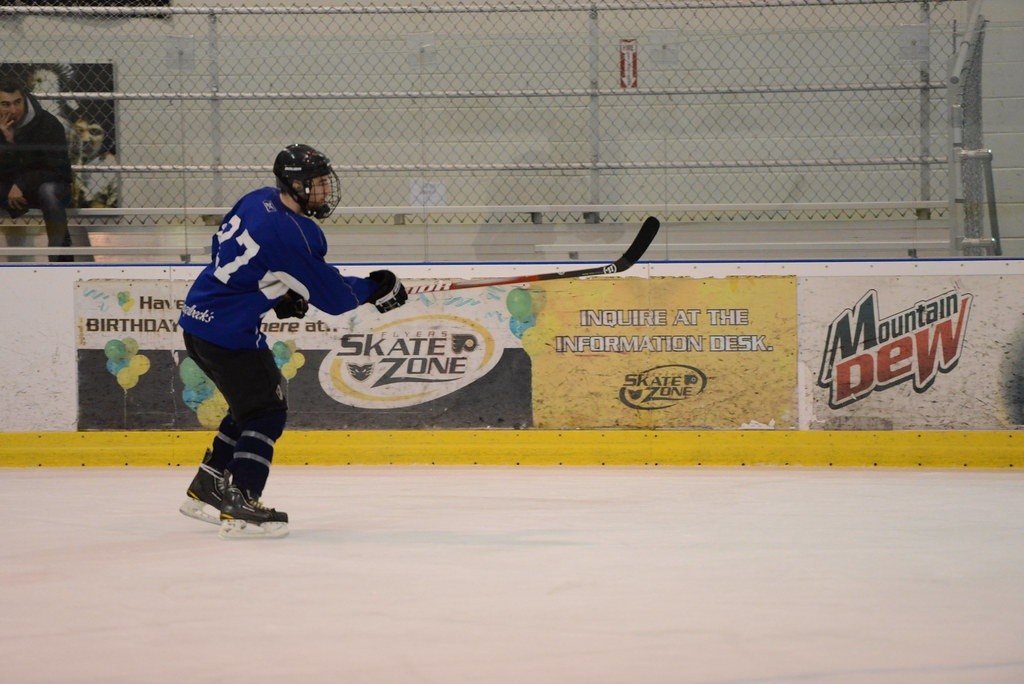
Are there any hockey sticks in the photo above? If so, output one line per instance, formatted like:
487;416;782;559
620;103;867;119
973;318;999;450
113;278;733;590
395;215;662;295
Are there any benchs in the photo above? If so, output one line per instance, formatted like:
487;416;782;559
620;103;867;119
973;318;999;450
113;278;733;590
0;199;963;260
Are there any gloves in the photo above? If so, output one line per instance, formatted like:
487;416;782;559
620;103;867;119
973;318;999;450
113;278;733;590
272;290;308;319
366;270;407;313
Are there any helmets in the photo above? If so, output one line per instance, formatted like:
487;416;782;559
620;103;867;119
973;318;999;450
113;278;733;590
273;143;342;219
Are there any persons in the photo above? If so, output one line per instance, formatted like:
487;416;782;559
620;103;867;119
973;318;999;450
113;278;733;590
178;144;408;539
69;113;119;208
0;77;75;262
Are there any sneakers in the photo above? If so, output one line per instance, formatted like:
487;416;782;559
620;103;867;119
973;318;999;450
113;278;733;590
220;470;290;539
179;447;224;525
